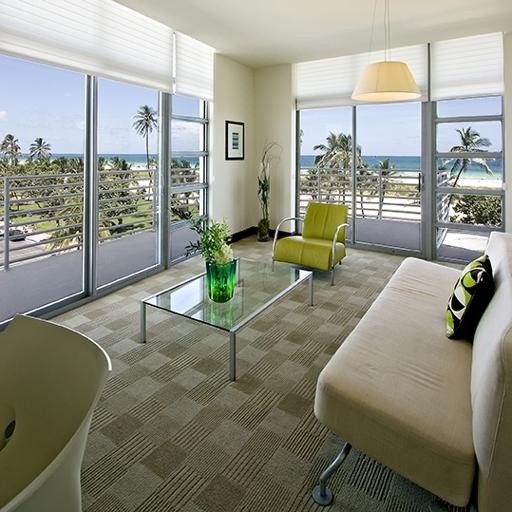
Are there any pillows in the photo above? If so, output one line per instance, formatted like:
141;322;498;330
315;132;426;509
443;254;494;339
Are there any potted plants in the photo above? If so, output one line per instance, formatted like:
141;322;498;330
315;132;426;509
256;139;282;242
184;215;241;303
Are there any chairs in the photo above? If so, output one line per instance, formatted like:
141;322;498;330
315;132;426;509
0;312;113;512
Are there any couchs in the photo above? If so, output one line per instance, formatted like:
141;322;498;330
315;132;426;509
311;229;512;512
271;203;351;286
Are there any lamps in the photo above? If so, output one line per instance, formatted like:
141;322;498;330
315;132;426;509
351;1;422;102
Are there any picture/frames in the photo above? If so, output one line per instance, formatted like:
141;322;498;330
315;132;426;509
225;120;246;160
